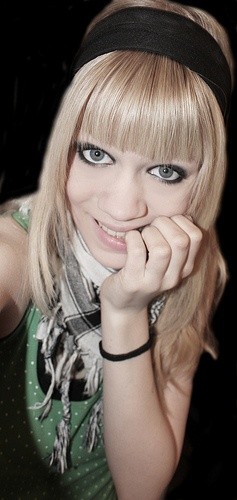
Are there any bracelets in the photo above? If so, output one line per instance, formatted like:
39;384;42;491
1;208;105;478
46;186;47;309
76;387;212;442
99;325;156;361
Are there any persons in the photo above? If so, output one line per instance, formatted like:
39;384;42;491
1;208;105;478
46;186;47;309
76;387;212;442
0;1;234;498
